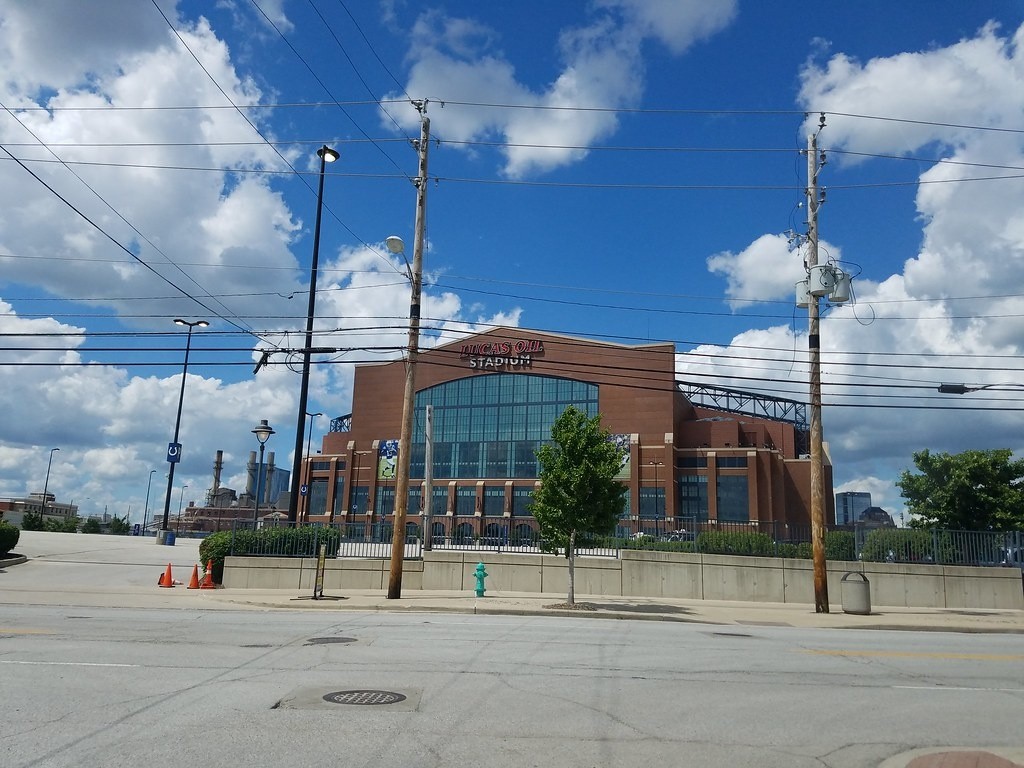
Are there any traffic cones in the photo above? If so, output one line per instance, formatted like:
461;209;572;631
201;560;215;589
157;572;184;585
159;563;175;588
187;564;200;589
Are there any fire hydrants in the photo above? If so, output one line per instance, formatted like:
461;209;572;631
472;562;488;597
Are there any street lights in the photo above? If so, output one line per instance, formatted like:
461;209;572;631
251;420;275;531
142;470;156;535
177;486;188;537
40;448;60;521
160;319;210;531
300;412;322;525
386;237;421;599
848;492;858;530
649;461;663;537
353;452;367;523
288;145;340;529
218;495;227;531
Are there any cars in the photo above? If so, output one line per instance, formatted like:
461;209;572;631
858;552;864;559
886;550;908;564
980;546;1024;573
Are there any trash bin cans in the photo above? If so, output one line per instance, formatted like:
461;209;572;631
165;532;175;546
840;570;871;614
